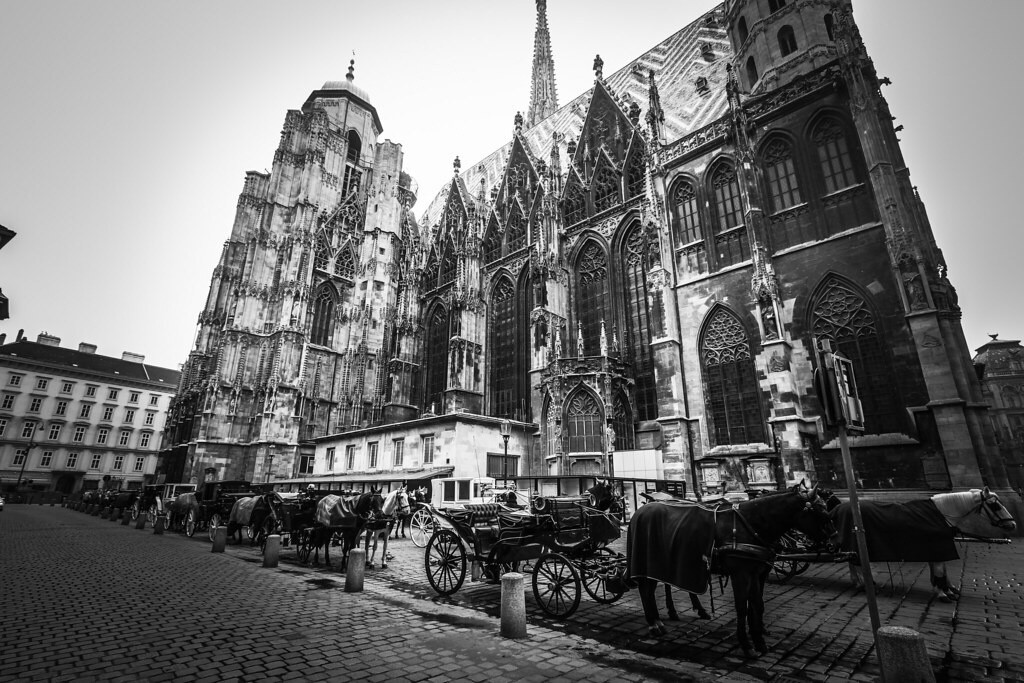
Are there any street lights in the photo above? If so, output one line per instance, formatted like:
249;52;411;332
500;410;513;488
17;420;45;487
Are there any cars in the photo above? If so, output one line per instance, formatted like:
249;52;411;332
0;489;24;511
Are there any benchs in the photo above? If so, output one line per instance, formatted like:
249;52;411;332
461;503;501;537
530;493;591;527
276;492;310;502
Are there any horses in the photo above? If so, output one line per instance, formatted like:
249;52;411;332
627;477;842;663
170;483;427;576
827;486;1016;602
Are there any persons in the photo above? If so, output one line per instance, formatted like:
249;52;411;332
88;487;112;513
607;424;615;452
598;483;613;519
555;424;562;451
506;484;517;505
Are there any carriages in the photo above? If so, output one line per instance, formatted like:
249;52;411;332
425;477;838;659
82;471;428;573
695;436;1017;603
408;475;622;559
